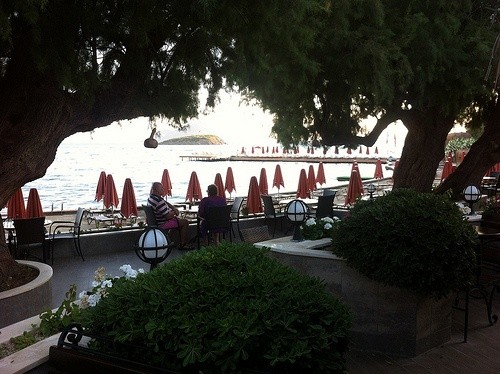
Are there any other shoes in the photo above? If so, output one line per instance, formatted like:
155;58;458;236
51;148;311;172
177;242;192;249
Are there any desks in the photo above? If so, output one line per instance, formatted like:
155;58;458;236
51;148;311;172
2;218;52;256
456;177;500;260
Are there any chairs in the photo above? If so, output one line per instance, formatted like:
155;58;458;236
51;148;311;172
461;264;500;343
13;186;336;268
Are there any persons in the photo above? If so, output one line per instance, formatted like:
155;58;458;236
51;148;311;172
147;182;193;250
198;184;227;246
392;160;400;189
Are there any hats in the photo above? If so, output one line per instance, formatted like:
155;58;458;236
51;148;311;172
206;184;217;192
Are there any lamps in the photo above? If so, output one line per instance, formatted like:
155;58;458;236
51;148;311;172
367;183;376;198
283;199;310;242
134;227;174;271
462;184;482;215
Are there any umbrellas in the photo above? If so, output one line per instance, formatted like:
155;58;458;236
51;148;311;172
120;179;138;219
241;146;378;159
296;162;325;199
225;167;236;199
373;158;384;186
247;176;263;218
273;165;284;196
26;189;43;218
214;173;226;202
160;169;172;201
186;171;203;217
95;171;119;225
441;146;500;184
258;167;269;196
344;160;364;207
7;186;26;219
